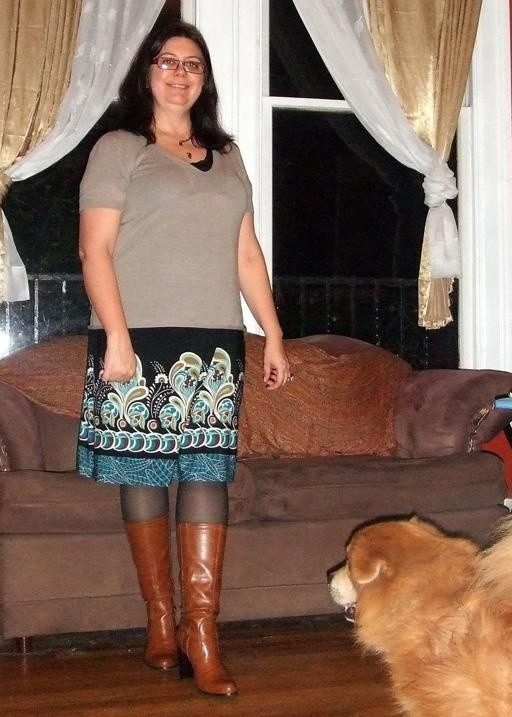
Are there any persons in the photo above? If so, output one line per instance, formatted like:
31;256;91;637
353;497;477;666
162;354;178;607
76;22;293;698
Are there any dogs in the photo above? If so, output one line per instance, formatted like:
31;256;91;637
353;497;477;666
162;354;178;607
326;512;512;716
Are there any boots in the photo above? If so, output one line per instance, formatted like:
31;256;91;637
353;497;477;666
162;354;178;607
171;522;237;696
125;515;178;671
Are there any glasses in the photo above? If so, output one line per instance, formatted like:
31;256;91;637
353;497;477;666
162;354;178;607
149;56;207;74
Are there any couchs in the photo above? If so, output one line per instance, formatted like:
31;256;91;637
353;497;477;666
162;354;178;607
0;332;512;652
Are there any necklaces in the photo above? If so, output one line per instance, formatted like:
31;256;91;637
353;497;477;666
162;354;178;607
150;118;201;158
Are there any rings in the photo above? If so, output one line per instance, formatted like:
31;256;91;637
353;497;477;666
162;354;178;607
286;376;292;380
